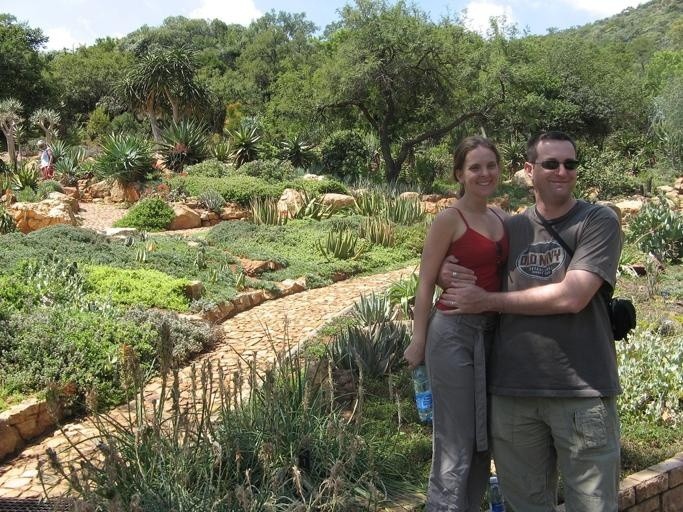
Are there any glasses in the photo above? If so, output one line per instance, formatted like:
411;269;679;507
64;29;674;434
529;160;579;170
496;241;505;275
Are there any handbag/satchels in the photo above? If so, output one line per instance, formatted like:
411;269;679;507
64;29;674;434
607;298;636;340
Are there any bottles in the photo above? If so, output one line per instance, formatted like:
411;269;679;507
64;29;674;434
412;364;433;423
487;476;507;512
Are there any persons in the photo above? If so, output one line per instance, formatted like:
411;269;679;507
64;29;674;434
36;140;53;180
